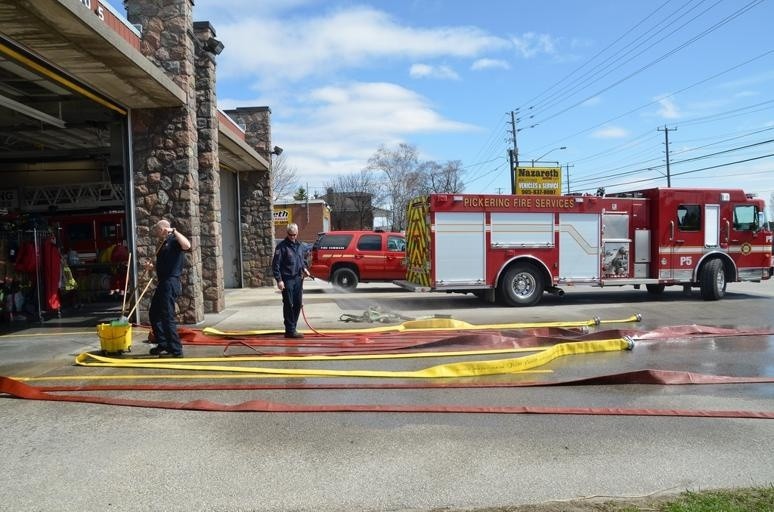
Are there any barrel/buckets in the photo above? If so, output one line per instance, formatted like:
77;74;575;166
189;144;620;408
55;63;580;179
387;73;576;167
97;322;132;356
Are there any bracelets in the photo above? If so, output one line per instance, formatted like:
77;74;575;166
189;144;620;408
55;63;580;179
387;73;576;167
173;227;176;234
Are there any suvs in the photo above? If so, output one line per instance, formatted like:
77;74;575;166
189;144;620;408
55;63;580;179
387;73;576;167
308;230;405;291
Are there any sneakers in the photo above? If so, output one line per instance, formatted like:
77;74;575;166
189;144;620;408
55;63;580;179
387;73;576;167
160;350;183;357
150;347;162;355
285;332;304;337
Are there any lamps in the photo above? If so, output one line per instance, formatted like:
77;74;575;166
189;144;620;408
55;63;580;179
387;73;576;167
202;37;224;56
267;145;282;156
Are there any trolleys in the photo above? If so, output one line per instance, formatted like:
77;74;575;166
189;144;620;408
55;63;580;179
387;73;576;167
95;319;134;358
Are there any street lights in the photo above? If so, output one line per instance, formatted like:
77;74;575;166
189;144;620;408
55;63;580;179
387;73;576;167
647;168;671;188
531;146;568;167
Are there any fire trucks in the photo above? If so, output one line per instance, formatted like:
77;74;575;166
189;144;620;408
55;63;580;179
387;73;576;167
0;183;128;301
404;187;774;307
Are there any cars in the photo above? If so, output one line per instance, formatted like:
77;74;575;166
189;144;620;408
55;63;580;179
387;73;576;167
275;238;311;259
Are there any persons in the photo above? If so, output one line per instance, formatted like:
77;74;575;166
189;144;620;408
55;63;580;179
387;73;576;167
144;220;191;357
272;223;311;338
